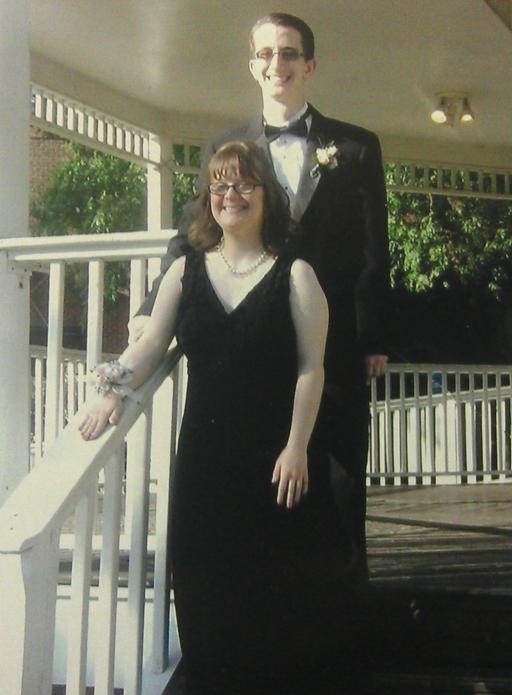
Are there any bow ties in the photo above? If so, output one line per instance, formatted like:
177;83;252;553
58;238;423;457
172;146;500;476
264;120;307;143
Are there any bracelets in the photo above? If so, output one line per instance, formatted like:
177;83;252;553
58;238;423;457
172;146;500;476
85;359;134;401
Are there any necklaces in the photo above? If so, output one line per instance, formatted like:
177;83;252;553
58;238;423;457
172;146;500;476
218;237;268;276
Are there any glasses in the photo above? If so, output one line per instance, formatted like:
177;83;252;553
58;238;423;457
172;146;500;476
209;182;266;196
256;47;304;59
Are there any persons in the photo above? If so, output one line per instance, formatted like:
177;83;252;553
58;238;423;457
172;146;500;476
78;137;330;695
125;12;391;669
